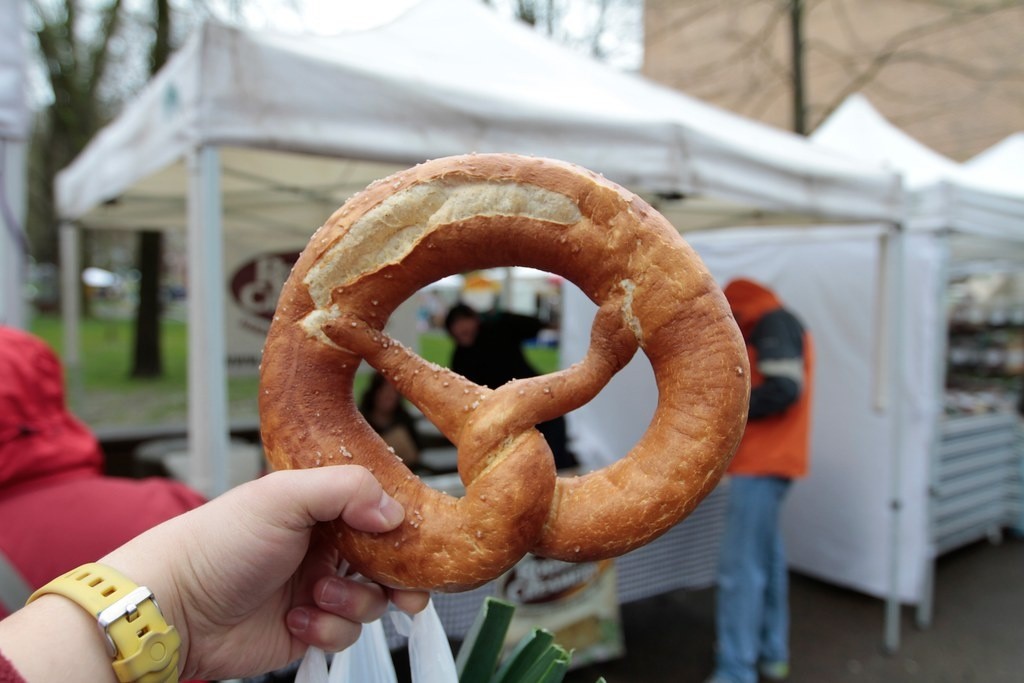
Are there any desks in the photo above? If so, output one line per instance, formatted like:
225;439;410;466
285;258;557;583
379;473;735;648
930;416;1024;557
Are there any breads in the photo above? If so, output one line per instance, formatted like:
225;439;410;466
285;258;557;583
259;151;749;591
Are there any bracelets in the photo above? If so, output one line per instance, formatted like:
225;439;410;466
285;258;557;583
25;563;181;683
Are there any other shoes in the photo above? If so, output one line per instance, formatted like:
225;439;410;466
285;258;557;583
757;657;790;679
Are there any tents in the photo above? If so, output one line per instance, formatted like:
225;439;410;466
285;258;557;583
54;0;904;655
678;89;1024;628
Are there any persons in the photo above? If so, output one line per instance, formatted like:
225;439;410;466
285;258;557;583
361;370;425;473
0;326;212;683
441;302;568;471
706;278;813;683
0;464;429;683
